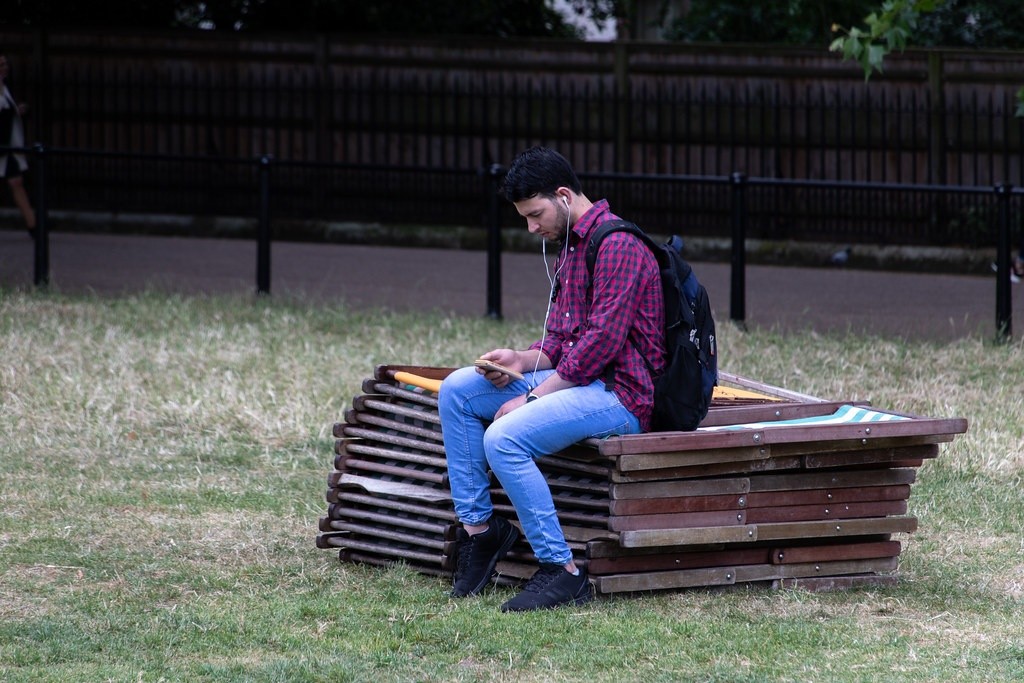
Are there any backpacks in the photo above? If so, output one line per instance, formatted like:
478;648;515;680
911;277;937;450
555;221;718;433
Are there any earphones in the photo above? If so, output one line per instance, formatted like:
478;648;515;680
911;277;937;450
562;196;569;208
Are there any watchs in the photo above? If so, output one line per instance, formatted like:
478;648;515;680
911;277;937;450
525;389;539;403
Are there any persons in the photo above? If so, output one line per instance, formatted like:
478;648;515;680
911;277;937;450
439;145;665;613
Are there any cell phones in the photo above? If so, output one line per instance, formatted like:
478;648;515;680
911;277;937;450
474;360;524;380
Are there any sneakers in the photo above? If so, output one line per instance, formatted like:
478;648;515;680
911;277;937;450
450;515;519;599
499;562;589;613
